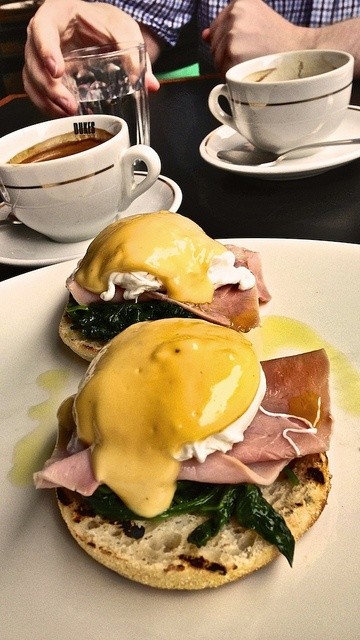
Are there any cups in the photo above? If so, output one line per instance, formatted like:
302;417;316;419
0;113;160;242
60;41;152;166
206;48;355;161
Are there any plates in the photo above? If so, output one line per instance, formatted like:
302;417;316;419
197;102;360;176
0;239;360;637
0;169;183;264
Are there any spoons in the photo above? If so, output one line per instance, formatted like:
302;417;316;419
217;138;360;166
0;207;28;228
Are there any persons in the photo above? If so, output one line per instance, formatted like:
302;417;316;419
22;0;360;120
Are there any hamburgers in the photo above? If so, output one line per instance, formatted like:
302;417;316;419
32;316;334;591
58;210;272;363
